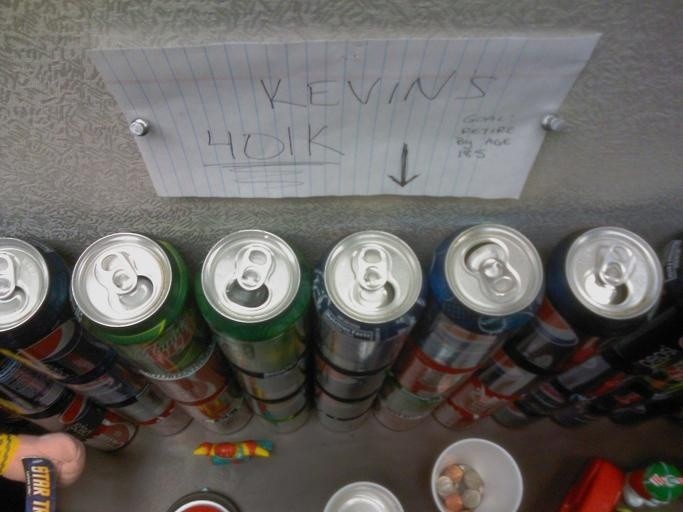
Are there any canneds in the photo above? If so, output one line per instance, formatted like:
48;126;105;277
0;221;683;452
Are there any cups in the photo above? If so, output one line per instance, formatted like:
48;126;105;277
322;482;404;512
426;437;524;512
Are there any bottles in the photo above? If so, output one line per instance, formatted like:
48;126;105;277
612;459;682;512
